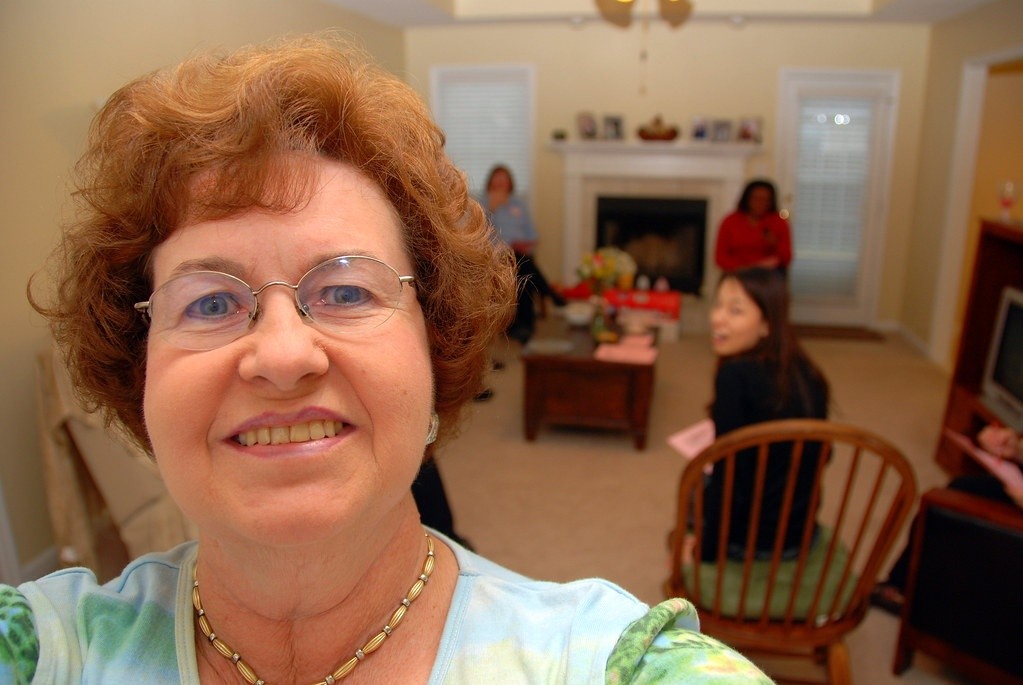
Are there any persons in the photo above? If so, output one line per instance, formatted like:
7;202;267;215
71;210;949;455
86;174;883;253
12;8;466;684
874;424;1023;616
0;39;774;685
409;458;474;552
480;165;567;346
714;180;793;285
668;268;832;564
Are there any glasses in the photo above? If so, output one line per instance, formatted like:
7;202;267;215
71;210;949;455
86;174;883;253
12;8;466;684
135;255;419;352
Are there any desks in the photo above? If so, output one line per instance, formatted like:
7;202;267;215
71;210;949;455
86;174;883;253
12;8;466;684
517;318;659;451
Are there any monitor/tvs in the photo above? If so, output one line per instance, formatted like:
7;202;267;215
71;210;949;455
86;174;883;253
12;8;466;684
980;286;1023;438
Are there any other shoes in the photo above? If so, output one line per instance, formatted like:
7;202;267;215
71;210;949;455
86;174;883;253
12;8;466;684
868;581;904;617
473;386;493;403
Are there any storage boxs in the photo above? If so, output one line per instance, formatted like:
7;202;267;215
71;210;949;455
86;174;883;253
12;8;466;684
602;289;682;341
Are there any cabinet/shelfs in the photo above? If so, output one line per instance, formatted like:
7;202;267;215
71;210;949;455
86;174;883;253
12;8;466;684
931;217;1023;478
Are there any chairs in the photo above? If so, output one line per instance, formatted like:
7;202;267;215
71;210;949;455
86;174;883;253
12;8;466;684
891;474;1023;685
664;420;917;685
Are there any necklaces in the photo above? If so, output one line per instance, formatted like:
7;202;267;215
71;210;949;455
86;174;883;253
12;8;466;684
192;532;435;685
